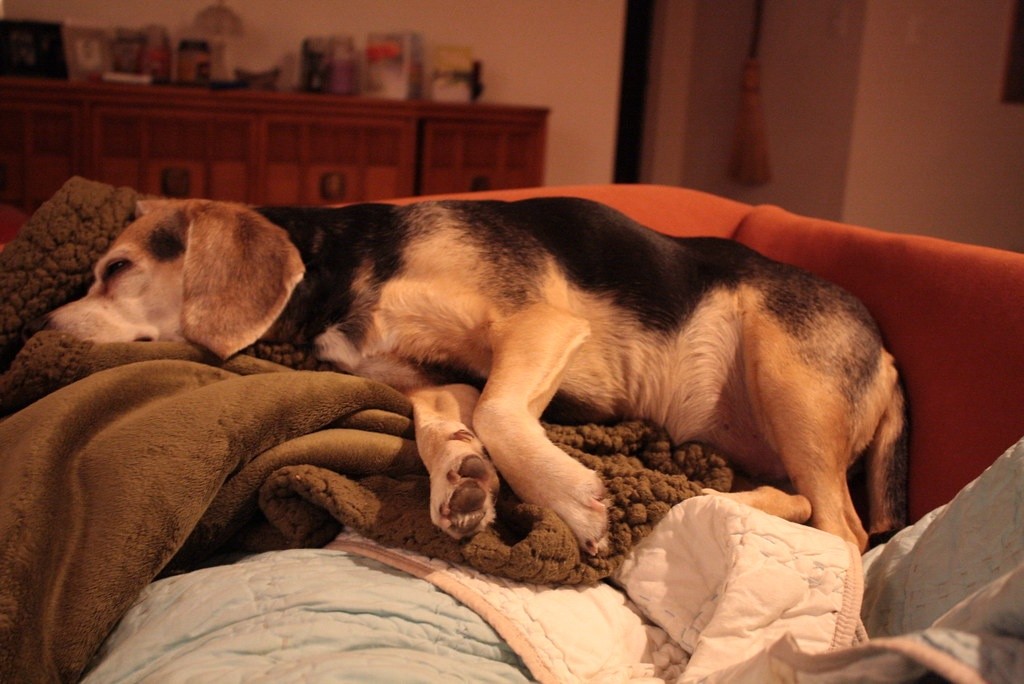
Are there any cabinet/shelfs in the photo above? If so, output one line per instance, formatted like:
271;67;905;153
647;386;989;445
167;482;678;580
0;77;551;214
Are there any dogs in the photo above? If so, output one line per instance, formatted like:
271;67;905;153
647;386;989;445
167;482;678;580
21;196;910;557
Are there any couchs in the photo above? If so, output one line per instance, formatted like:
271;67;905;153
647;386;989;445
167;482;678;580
0;183;1024;684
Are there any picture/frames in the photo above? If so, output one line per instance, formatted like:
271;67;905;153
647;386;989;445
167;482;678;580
63;25;114;81
